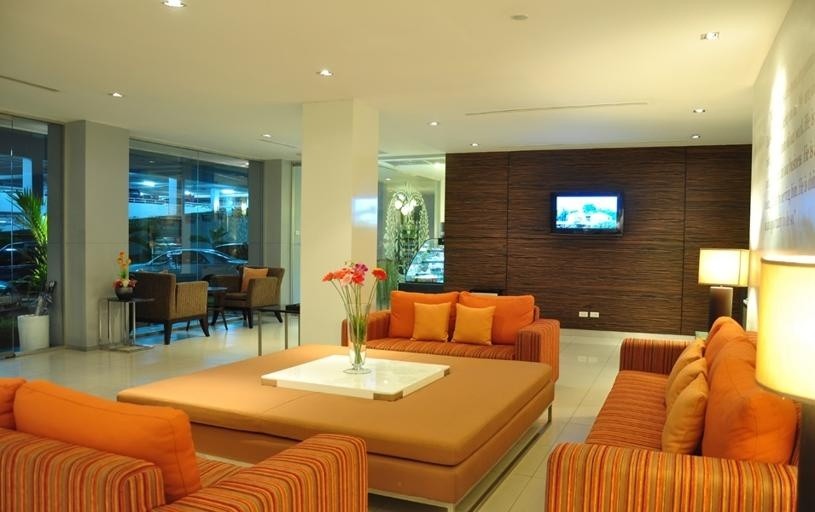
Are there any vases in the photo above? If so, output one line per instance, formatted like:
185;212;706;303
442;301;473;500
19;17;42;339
115;286;133;301
342;302;374;375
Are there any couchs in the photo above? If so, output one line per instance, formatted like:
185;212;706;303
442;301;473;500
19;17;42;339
545;313;802;512
0;376;368;512
341;288;560;386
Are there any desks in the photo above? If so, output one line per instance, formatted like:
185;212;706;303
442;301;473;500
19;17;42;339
96;296;157;353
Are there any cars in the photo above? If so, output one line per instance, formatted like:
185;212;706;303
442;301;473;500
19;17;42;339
0;281;24;316
128;246;248;290
216;241;247;259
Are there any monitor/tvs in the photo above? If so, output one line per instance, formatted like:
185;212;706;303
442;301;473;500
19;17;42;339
550;190;625;237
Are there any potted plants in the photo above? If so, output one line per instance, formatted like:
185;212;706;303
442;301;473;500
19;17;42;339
1;185;57;353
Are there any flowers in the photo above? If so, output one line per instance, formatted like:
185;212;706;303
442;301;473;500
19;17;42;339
112;249;136;288
322;262;389;365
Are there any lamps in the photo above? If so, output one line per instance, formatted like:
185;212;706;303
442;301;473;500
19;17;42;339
753;252;815;407
694;247;749;332
382;187;431;273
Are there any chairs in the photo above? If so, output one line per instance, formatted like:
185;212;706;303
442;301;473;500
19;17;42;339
129;271;209;345
207;264;285;329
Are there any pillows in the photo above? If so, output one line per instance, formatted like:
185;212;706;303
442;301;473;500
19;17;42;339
411;301;452;343
449;302;497;346
663;334;708;457
240;267;268;292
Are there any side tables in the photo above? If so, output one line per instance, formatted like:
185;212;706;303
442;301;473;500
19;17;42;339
185;286;229;332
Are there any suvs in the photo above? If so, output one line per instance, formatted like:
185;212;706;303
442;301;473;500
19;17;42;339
0;240;45;294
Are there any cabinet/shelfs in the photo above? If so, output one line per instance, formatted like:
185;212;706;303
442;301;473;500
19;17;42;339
404;237;444;285
